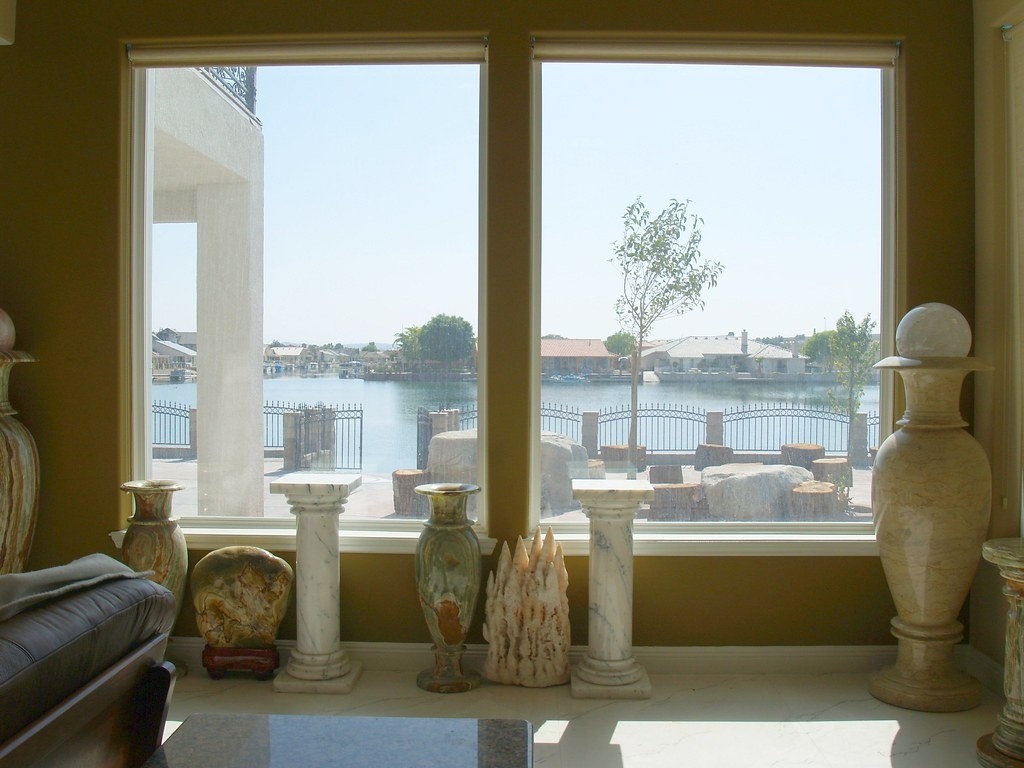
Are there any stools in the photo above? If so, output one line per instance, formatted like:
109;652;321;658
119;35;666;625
392;469;431;515
793;481;839;523
813;457;852;486
601;445;646;473
647;483;709;522
589;459;604;478
694;443;733;470
781;444;824;471
650;466;684;483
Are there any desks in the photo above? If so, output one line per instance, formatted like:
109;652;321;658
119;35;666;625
141;713;534;768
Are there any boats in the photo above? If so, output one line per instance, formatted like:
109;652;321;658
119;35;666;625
653;357;738;383
541;374;591;384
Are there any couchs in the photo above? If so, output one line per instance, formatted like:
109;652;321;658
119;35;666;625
0;553;177;768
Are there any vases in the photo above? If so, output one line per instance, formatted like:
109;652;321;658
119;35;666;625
415;483;482;693
120;480;188;681
